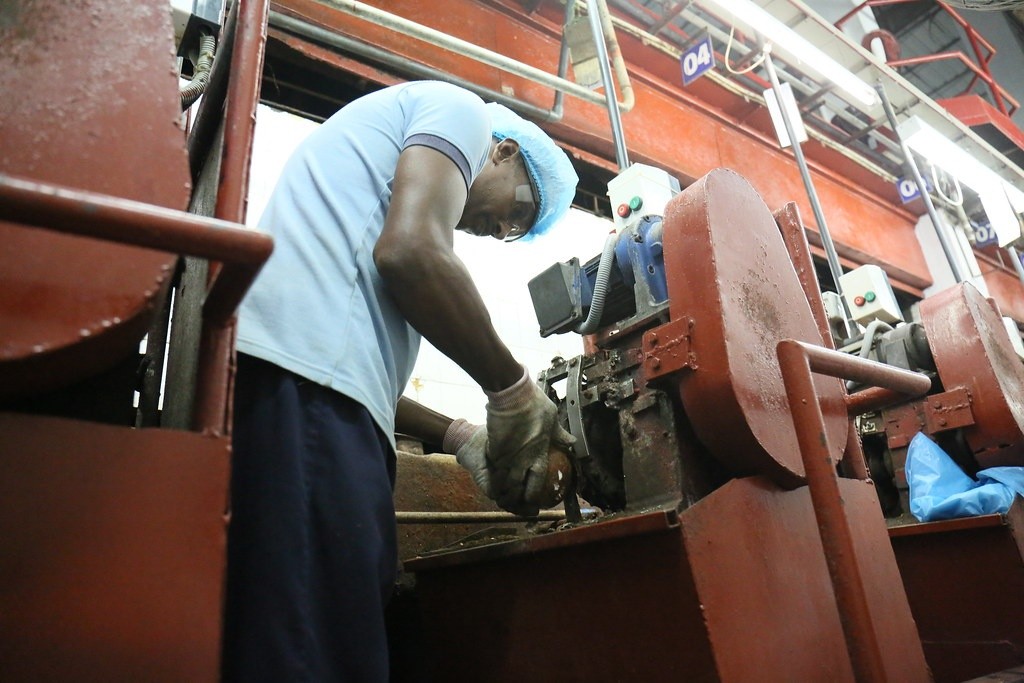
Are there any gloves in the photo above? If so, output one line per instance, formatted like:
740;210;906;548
442;417;496;502
483;362;577;516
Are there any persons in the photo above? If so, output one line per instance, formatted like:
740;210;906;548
224;80;578;682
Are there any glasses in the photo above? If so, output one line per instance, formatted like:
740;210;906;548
503;155;538;243
